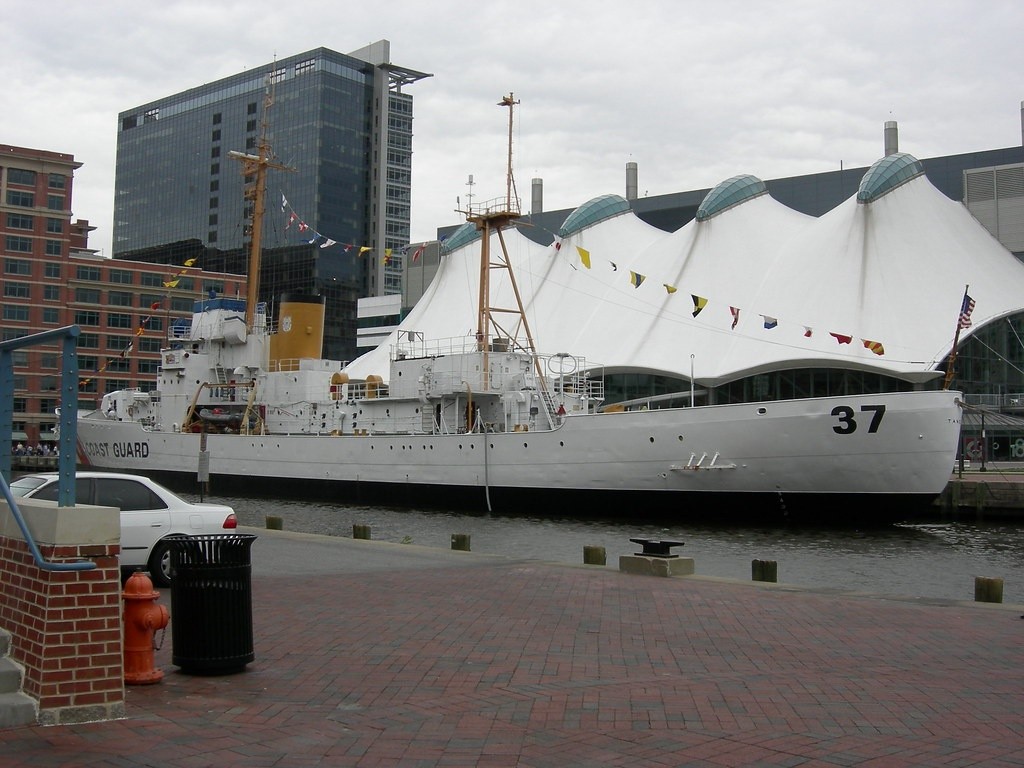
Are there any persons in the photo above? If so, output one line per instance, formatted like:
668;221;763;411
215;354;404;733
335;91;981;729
17;441;58;457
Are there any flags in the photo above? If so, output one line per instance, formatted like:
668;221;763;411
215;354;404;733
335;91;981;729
957;294;976;330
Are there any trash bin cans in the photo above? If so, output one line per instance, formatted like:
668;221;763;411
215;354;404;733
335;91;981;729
160;534;258;677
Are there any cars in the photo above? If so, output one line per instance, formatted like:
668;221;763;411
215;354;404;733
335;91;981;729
9;470;237;589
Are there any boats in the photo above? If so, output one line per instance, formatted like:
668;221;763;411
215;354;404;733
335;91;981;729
54;92;1024;532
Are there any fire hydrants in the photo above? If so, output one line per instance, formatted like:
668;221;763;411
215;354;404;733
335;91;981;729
121;567;171;686
